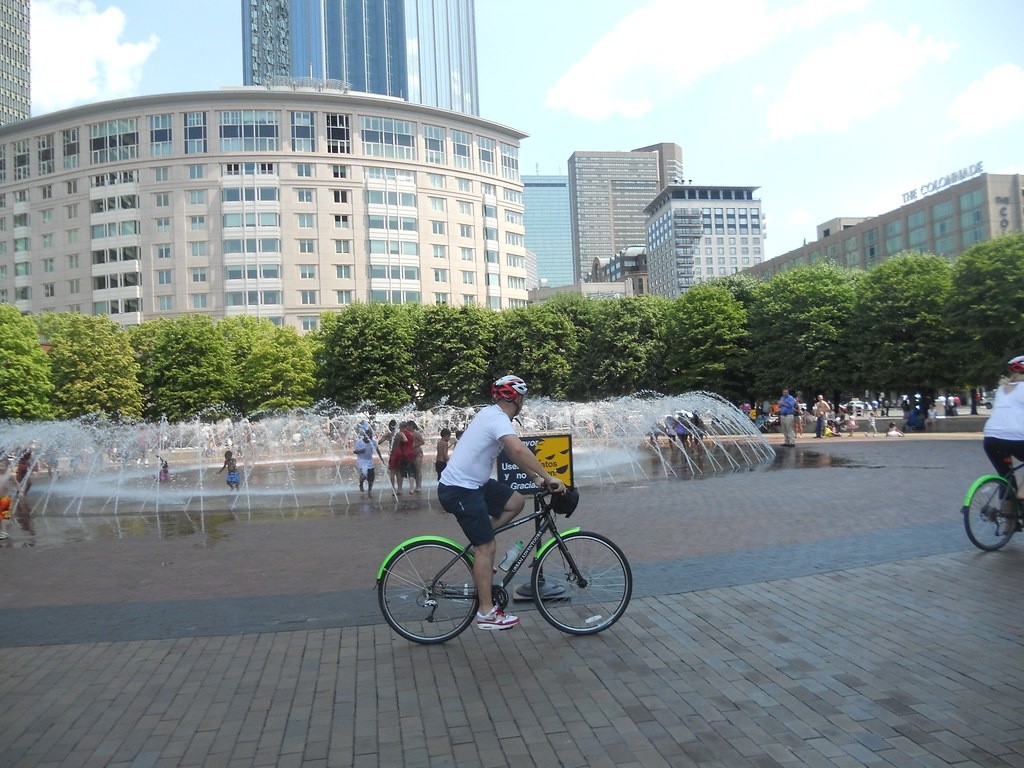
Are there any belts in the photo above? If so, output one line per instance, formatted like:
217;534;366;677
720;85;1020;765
779;412;792;416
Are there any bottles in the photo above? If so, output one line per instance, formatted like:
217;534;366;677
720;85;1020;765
498;540;526;572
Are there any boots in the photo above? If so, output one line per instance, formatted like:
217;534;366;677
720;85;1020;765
995;497;1022;535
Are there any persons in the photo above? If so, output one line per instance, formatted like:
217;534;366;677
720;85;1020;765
983;356;1024;534
352;418;424;497
649;411;704;449
435;429;450;481
0;443;84;547
777;389;796;447
454;430;464;448
218;450;240;491
438;375;566;631
740;395;937;439
154;460;170;482
938;393;961;416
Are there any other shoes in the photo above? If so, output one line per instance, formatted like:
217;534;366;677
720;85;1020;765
0;531;10;539
788;444;795;446
780;444;786;446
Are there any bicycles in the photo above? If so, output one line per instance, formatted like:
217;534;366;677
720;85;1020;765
374;481;633;644
960;449;1024;551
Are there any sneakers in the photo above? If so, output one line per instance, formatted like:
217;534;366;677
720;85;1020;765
466;544;497;575
476;604;519;630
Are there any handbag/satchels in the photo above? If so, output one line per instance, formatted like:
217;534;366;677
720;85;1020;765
400;462;417;478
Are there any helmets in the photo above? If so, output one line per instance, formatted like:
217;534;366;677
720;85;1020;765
1006;355;1024;375
490;374;528;402
